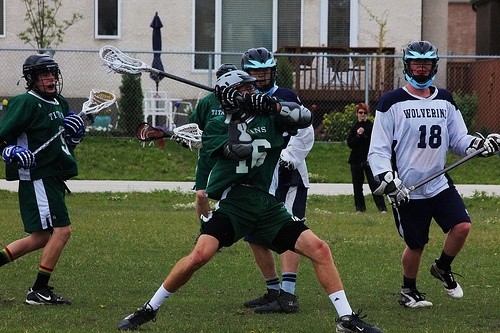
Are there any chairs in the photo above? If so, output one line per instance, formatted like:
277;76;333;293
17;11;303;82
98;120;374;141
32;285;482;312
115;91;201;133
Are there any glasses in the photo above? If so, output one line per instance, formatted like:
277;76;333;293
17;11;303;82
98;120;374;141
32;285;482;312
357;111;368;115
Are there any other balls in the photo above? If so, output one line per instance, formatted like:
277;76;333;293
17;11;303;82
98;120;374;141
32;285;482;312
113;60;122;68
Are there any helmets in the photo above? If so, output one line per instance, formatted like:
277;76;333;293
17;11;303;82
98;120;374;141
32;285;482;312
242;47;277;92
216;63;236;78
23;54;64;101
215;72;257;88
403;40;439;90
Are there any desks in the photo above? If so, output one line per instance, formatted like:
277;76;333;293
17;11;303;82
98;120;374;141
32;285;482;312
141;98;183;130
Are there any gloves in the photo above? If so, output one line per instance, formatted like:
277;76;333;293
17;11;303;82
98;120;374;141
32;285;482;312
2;145;36;170
244;93;277;116
372;170;410;208
62;110;85;144
465;131;500;158
218;88;242;113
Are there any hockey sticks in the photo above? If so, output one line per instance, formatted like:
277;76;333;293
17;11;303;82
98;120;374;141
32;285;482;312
12;88;117;171
100;45;243;103
170;133;202;149
136;121;175;142
406;146;488;192
174;124;203;137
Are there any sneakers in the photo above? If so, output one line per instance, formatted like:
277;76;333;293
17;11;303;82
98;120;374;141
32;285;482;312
335;308;383;333
118;300;160;330
254;288;299;314
26;284;71;306
430;258;463;299
398;286;433;308
243;292;279;307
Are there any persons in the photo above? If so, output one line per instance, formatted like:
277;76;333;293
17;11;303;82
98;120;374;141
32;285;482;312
346;103;387;214
0;54;85;305
178;62;238;252
240;47;314;315
310;104;323;141
117;69;384;333
367;41;500;307
